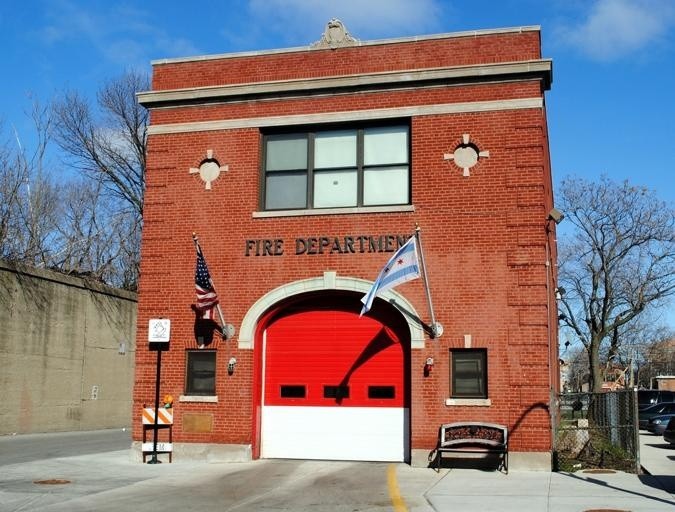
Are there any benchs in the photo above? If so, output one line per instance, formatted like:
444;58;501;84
430;420;510;475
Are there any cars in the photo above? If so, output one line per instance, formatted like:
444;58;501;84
638;389;674;444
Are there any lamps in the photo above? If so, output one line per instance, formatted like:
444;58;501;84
228;358;237;372
547;208;565;233
426;357;434;371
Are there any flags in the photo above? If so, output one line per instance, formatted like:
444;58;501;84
192;235;221;321
358;231;421;319
